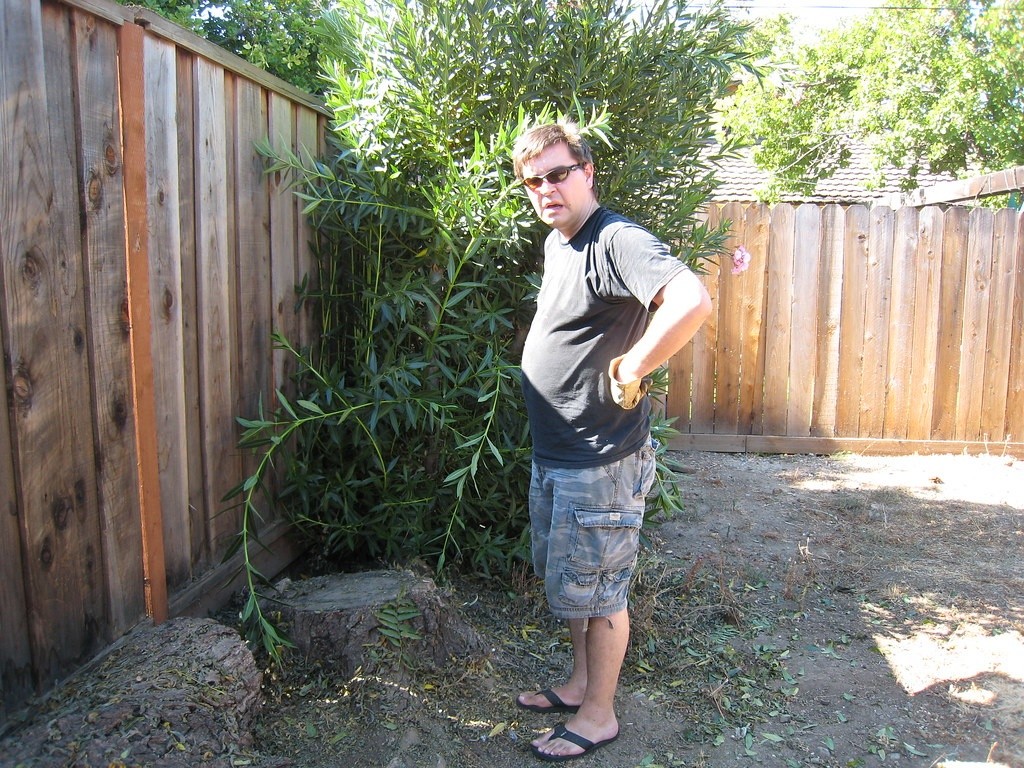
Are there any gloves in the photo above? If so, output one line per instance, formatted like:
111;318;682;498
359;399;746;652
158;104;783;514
608;354;654;410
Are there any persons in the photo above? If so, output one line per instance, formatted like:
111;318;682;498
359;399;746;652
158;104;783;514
513;121;711;760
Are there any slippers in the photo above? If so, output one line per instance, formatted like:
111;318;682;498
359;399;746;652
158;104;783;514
517;688;582;713
531;722;620;760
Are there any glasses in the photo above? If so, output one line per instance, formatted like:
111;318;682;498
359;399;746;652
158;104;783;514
523;161;584;189
